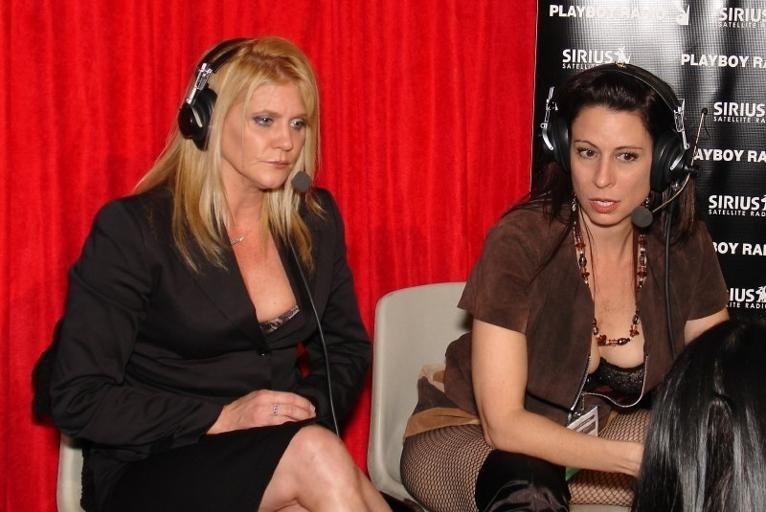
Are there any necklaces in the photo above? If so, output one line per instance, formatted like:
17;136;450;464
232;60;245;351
227;228;266;244
571;203;650;351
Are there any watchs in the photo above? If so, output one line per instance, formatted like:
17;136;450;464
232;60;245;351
631;313;766;512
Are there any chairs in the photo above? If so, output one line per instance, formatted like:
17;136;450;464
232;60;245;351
49;429;88;512
362;274;634;512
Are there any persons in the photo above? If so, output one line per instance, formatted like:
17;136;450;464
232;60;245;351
398;62;731;511
31;36;394;512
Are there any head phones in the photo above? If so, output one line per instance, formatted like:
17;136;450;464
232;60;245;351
539;61;702;193
179;37;255;151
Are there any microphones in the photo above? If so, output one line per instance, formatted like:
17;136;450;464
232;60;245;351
291;164;311;193
631;107;707;227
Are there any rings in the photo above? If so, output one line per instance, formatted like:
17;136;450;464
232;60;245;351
273;403;278;413
